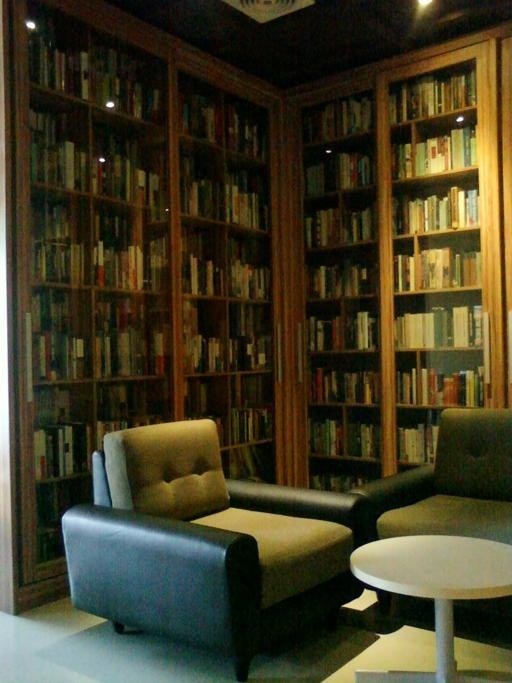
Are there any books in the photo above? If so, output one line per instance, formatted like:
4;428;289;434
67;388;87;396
177;70;268;157
29;190;165;292
35;385;171;482
29;19;164;122
302;96;380;493
387;68;484;466
180;140;269;230
35;480;93;561
183;298;275;374
186;407;274;483
25;93;167;209
182;236;271;299
30;286;168;382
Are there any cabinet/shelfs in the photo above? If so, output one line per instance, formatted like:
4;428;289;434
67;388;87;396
0;0;511;616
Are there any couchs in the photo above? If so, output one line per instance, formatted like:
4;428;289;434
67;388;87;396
59;415;378;682
348;407;511;635
